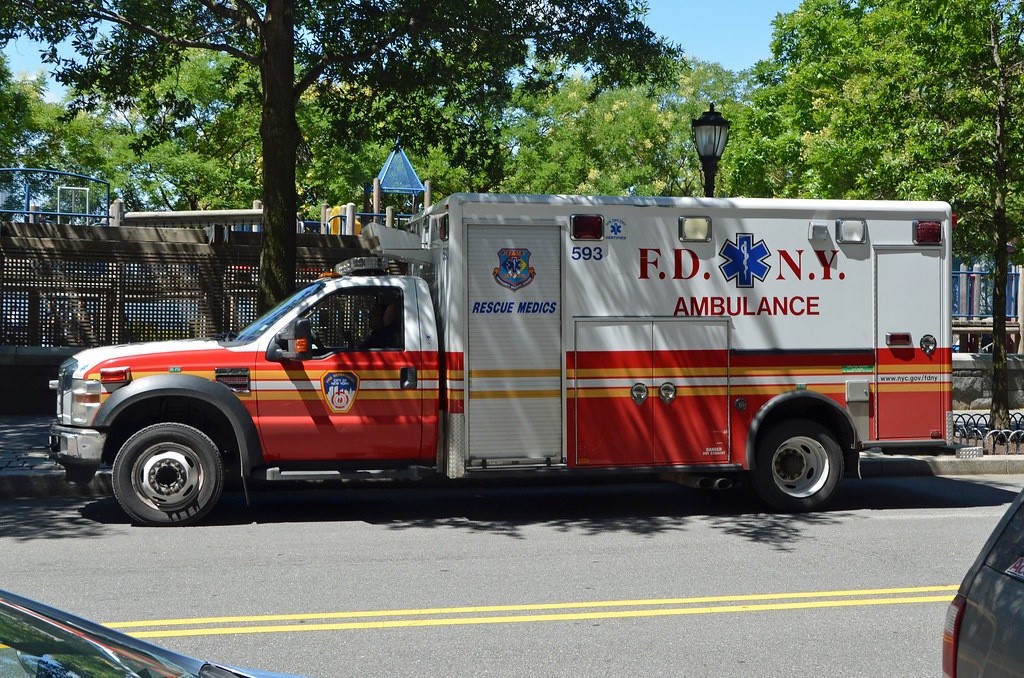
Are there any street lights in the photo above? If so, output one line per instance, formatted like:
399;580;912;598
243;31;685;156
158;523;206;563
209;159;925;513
692;104;732;198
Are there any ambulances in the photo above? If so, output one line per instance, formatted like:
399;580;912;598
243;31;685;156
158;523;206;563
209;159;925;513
45;194;958;528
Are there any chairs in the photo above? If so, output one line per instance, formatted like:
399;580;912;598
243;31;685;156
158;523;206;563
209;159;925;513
388;321;401;347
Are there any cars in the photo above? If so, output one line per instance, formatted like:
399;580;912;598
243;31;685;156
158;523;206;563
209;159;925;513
0;590;321;678
941;485;1023;677
